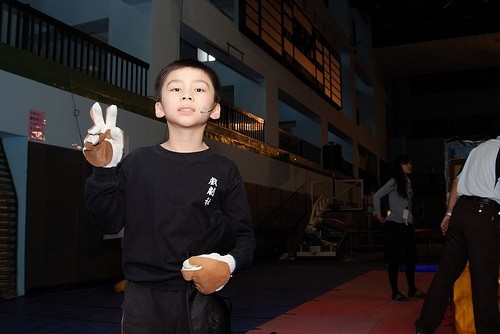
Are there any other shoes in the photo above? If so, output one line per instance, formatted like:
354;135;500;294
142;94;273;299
408;288;426;298
391;291;409;301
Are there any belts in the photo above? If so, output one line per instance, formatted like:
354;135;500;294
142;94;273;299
460;194;500;209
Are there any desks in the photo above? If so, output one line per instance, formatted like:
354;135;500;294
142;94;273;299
348;229;380;254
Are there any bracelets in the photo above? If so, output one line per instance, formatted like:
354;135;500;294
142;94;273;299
445;212;452;216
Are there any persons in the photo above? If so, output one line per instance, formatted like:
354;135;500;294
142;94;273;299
82;58;256;334
413;135;500;334
372;155;429;301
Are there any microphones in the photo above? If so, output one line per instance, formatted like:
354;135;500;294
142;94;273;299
200;102;217;114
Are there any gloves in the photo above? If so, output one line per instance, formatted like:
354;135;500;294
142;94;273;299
83;101;124;168
181;252;236;294
